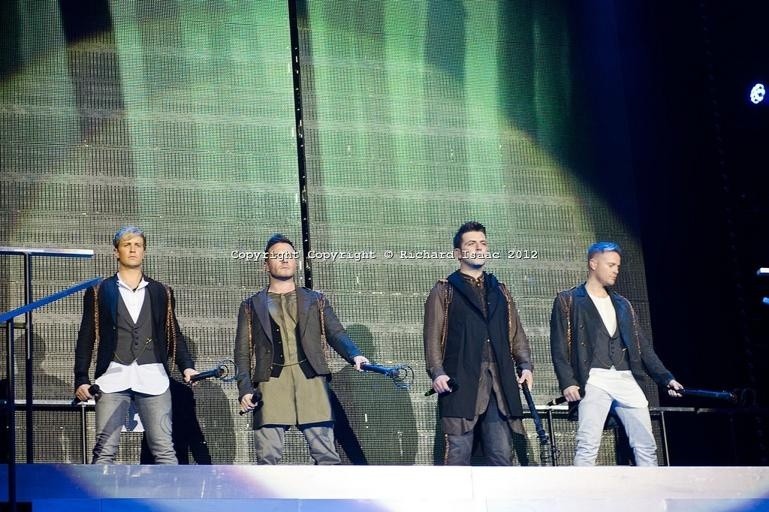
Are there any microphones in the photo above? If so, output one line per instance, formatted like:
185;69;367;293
424;378;455;396
547;389;585;406
239;392;262;416
71;384;99;408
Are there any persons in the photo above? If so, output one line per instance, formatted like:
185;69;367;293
234;234;370;465
549;240;686;467
423;222;533;466
74;226;200;466
